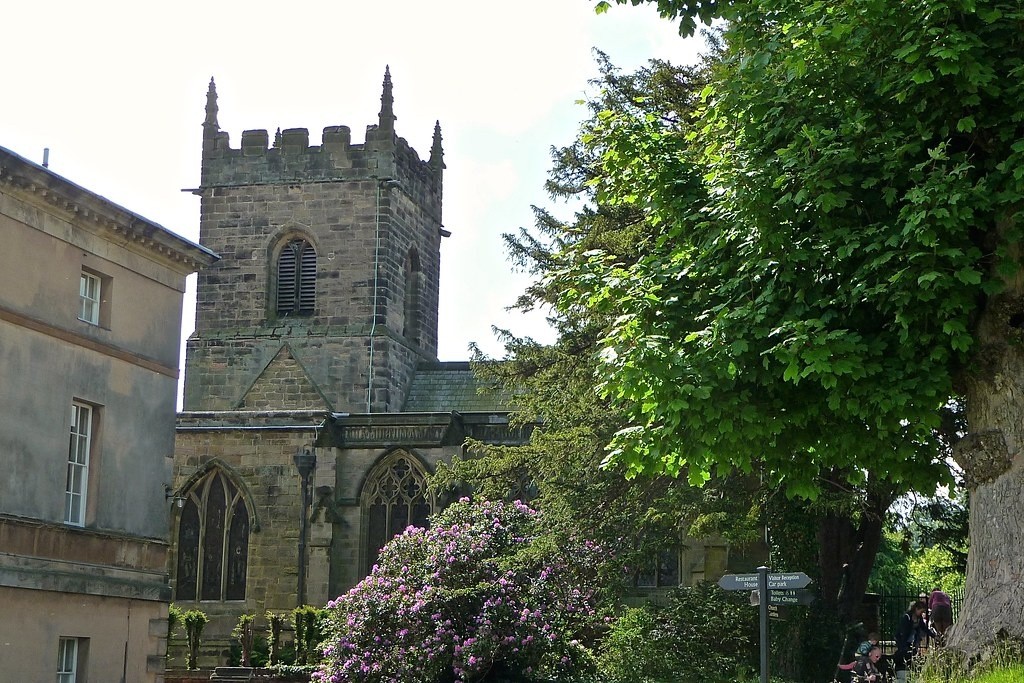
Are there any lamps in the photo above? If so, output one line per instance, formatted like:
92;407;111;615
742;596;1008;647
162;481;174;496
166;492;186;508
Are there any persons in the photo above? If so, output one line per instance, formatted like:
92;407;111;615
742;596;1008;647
850;588;954;683
